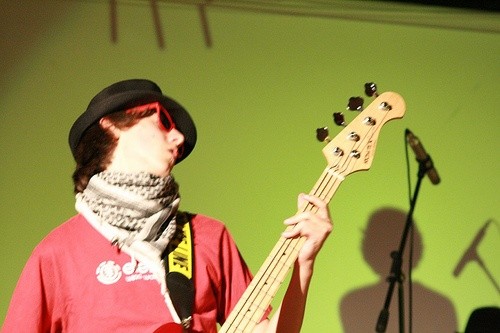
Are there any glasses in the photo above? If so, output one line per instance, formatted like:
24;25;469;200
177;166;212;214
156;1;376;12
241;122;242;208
115;101;185;158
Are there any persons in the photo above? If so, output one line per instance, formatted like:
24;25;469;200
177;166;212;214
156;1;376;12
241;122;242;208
1;80;333;333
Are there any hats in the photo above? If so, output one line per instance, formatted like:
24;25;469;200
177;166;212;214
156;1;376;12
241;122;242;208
68;79;197;165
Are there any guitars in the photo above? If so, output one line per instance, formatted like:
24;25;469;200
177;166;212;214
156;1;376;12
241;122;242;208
153;82;405;333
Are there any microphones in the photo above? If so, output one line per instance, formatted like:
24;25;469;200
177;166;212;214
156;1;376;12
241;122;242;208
407;129;441;184
